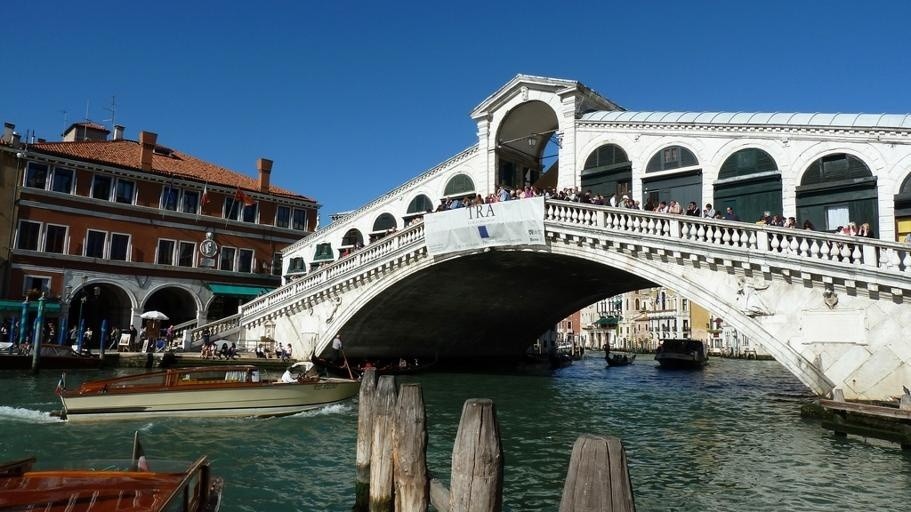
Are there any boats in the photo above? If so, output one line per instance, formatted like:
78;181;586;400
654;338;709;369
311;352;432;378
56;365;358;421
606;354;637;366
516;356;572;372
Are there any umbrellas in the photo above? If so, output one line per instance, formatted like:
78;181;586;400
140;310;171;330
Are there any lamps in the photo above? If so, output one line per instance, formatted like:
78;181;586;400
528;132;563;150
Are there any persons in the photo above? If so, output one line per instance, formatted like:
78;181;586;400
399;357;407;368
331;333;343;365
603;341;611;357
257;185;547;298
0;319;294;375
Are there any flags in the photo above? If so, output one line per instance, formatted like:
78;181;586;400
131;432;151;472
53;373;64;397
166;175;176;205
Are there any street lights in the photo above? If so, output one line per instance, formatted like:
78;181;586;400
65;273;100;354
